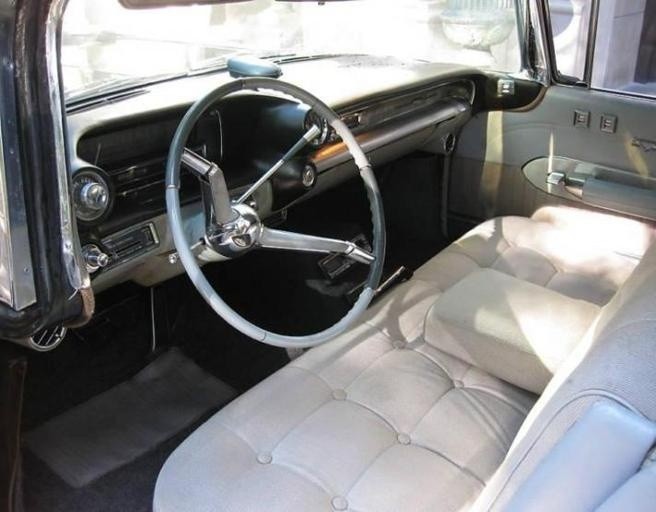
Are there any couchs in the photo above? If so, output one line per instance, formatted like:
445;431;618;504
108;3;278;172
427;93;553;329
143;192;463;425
142;205;656;512
